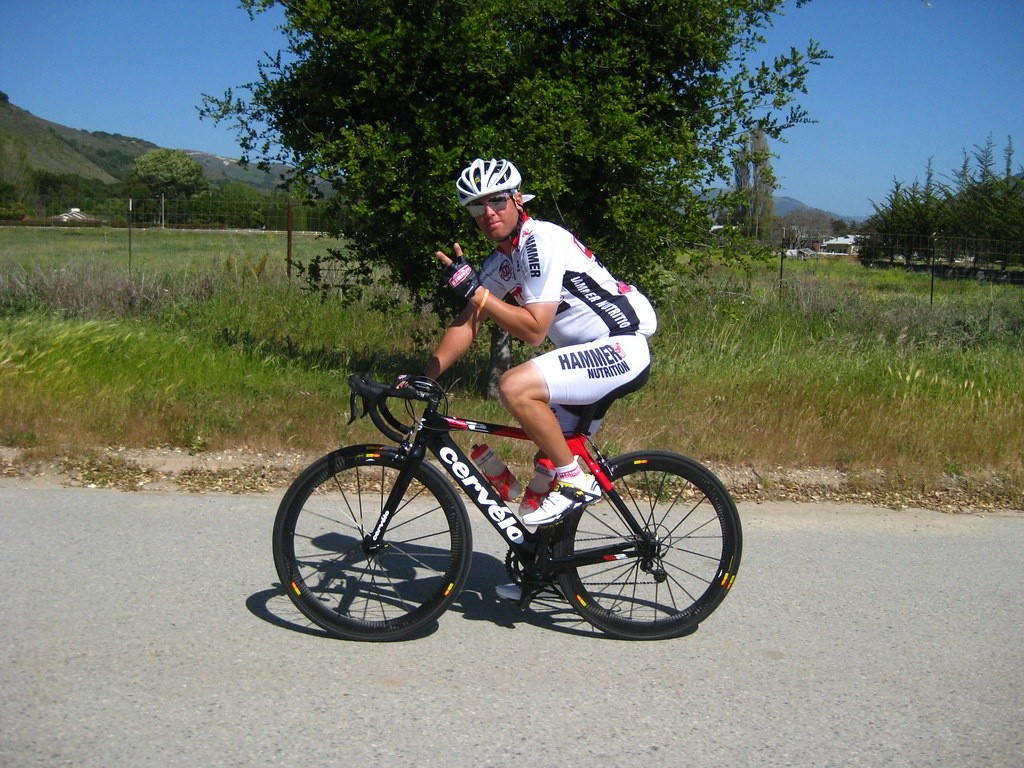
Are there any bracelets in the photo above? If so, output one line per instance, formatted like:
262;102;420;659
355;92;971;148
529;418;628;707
477;289;490;311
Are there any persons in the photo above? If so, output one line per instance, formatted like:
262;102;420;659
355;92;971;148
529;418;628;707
396;159;651;603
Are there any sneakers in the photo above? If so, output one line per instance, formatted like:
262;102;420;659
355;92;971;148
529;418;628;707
495;582;538;601
523;474;602;524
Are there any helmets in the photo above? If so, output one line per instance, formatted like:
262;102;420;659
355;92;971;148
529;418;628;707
455;158;522;207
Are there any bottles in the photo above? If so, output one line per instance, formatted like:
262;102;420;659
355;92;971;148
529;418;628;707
469;443;522;502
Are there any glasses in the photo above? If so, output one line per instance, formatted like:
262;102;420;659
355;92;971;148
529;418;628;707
466;194;513;218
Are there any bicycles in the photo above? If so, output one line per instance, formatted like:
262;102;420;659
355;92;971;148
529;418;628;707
270;367;745;644
